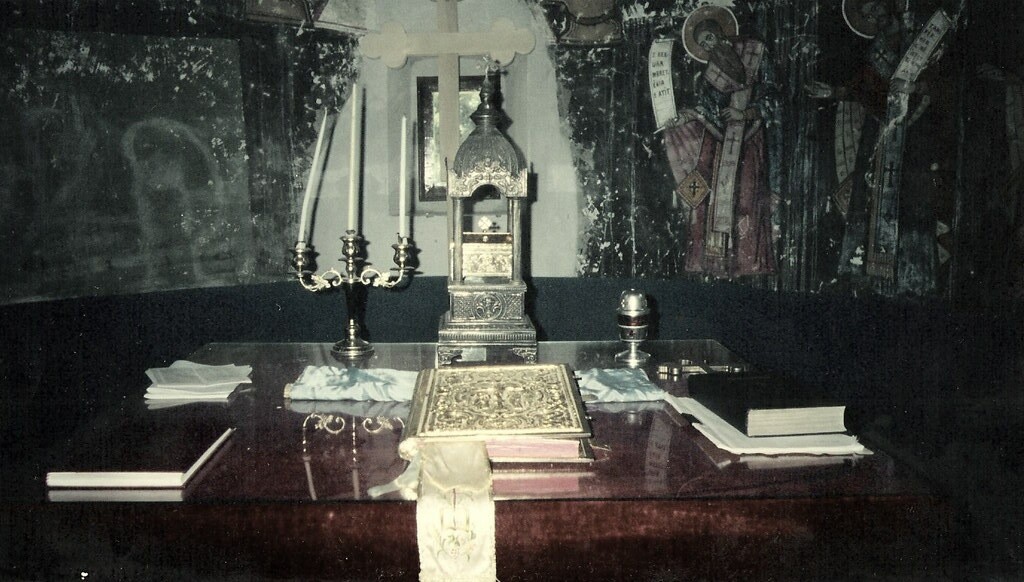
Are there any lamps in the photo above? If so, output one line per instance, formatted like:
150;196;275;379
436;51;538;365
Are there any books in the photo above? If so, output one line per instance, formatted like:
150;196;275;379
45;422;234;489
663;371;864;455
400;362;595;466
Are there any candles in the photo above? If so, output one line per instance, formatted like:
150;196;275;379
348;82;357;229
399;115;407;238
298;108;328;240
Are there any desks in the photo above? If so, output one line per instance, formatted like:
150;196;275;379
0;340;933;581
